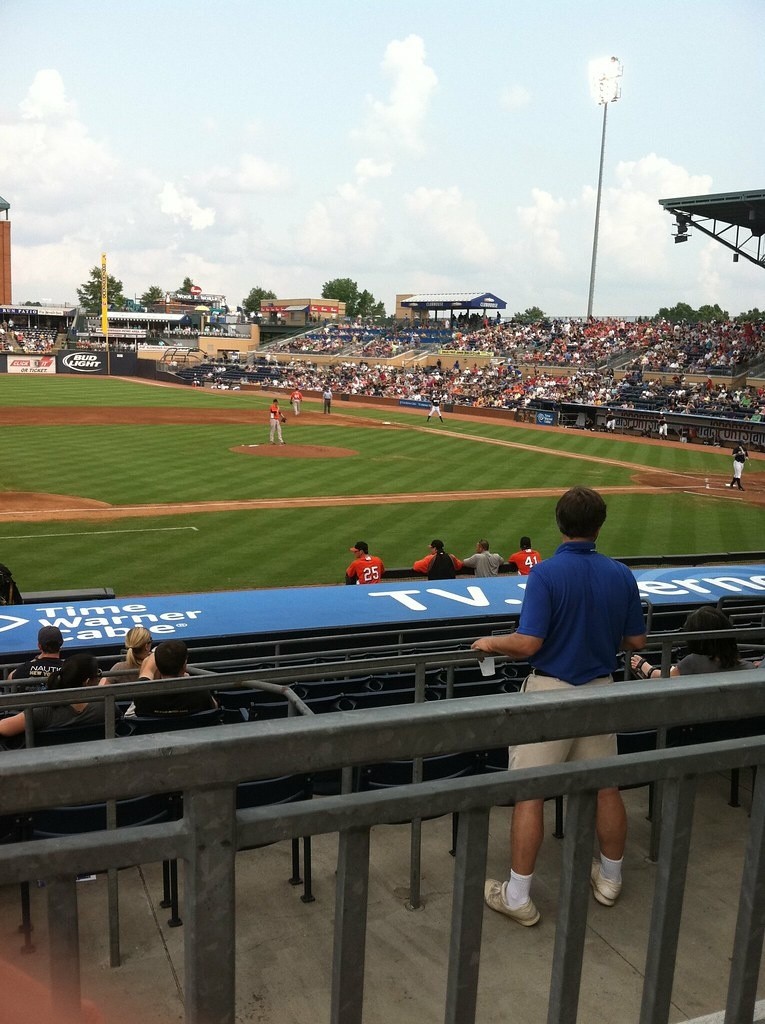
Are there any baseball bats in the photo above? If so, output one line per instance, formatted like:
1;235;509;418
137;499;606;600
739;446;751;466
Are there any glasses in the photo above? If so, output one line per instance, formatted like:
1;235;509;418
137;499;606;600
148;637;153;644
97;668;103;678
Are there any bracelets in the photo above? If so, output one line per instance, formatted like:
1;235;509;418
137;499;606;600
151;647;156;653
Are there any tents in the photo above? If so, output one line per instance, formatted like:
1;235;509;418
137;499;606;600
195;305;210;314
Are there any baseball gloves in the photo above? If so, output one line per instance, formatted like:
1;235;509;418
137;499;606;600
281;418;287;423
290;401;293;405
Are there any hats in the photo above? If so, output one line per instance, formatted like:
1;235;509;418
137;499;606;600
428;539;444;548
37;625;63;646
350;541;368;552
520;537;531;547
739;439;746;445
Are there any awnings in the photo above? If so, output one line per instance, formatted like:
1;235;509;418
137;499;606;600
284;304;309;312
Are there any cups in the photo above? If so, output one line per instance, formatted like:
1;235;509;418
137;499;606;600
479;657;496;676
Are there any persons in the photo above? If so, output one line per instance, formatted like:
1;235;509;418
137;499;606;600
269;398;287;445
461;539;504;579
630;606;756;679
605;409;615;433
754;658;765;669
0;319;198;354
447;312;765;452
194;312;453;404
290;388;302;417
730;438;749;491
472;490;646;926
344;542;385;586
0;626;218;737
426;393;443;424
509;536;542;577
323;388;332;414
413;536;464;580
656;412;668;440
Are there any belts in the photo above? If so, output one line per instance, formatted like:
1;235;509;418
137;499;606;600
529;667;610;678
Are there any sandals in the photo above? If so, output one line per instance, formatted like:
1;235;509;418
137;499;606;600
624;650;658;680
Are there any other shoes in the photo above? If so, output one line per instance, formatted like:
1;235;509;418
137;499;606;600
279;442;286;445
737;487;743;491
730;483;733;488
270;442;276;445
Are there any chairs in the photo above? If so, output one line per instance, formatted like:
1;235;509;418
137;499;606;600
176;364;282;382
606;386;756;419
14;326;58;346
0;590;765;957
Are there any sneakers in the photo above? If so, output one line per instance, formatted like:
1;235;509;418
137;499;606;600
590;856;623;906
484;878;540;927
